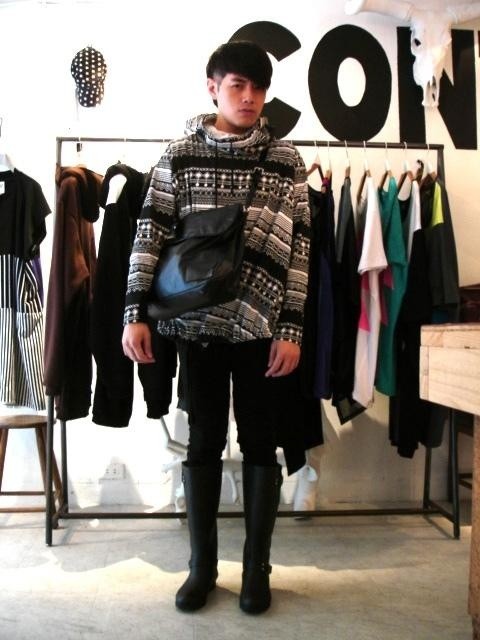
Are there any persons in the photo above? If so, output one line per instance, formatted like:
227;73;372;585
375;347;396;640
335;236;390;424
121;41;311;616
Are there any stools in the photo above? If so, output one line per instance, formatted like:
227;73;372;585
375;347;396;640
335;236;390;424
0;414;67;529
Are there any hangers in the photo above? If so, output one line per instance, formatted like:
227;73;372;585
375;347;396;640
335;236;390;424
0;112;15;173
71;134;438;191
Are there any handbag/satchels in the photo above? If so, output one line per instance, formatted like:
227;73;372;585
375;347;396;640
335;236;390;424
146;204;249;322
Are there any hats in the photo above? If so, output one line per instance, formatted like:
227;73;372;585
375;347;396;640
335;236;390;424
70;45;107;108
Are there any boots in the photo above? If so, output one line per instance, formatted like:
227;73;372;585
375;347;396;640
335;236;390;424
174;460;218;612
240;461;283;616
